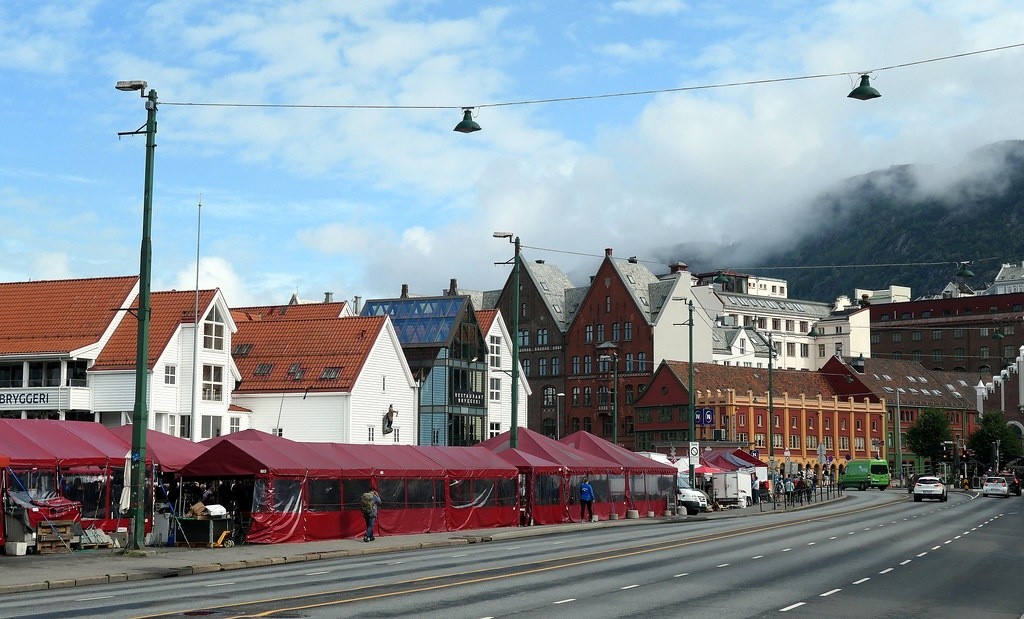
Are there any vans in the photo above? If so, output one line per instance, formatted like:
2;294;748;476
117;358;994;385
838;458;891;492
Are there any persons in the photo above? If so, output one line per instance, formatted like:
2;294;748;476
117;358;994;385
980;479;984;489
363;486;382;542
752;477;759;503
387;403;396;428
830;469;835;484
519;481;525;500
775;466;818;505
821;466;828;486
68;478;82;501
579;477;595;523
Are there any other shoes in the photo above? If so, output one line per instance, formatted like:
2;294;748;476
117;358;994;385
370;536;375;540
363;537;371;542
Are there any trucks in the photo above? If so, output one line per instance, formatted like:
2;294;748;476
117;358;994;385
592;452;708;515
711;472;753;509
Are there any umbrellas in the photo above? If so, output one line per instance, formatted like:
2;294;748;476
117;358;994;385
673;448;768;490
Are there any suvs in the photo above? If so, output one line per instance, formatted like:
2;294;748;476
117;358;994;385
997;472;1022;496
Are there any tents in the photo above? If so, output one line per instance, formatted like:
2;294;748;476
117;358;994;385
0;418;682;544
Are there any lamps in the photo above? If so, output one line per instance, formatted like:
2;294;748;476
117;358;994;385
713;268;728;284
955;261;974;277
847;74;881;100
991;327;1004;339
806;324;819;336
453;107;481;133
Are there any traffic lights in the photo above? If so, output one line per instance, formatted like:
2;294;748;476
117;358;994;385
1000;453;1004;464
943;451;952;461
963;450;968;462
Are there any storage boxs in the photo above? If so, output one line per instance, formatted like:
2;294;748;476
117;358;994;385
6;542;27;555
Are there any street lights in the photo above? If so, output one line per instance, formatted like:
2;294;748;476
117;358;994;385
599;352;619;448
731;323;778;478
115;79;162;557
672;296;698;490
492;230;523;451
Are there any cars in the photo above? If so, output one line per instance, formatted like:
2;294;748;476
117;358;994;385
907;473;933;493
913;476;948;502
982;476;1010;498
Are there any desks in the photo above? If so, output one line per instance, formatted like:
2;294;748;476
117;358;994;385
175;517;237;548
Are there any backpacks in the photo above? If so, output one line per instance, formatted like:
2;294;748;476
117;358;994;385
360;492;376;517
775;480;784;495
